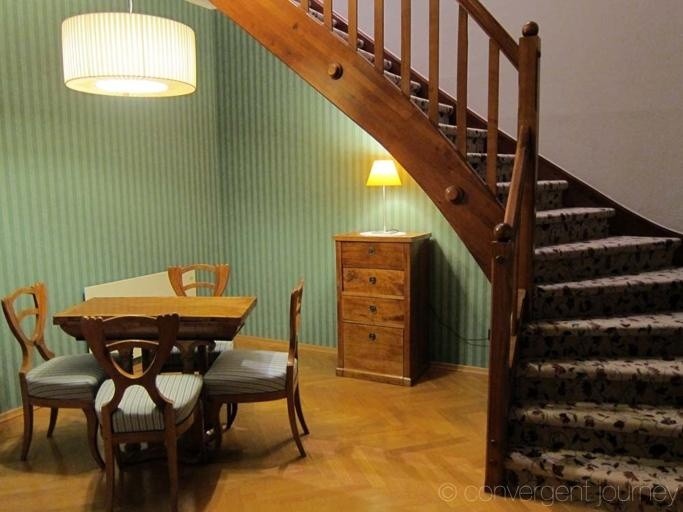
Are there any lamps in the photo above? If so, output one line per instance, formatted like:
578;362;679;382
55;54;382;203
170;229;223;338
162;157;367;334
367;160;402;233
61;0;197;98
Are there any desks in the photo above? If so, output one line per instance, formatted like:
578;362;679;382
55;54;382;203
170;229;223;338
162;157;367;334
52;296;258;466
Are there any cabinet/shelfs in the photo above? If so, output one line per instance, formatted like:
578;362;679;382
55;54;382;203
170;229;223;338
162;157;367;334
333;230;432;387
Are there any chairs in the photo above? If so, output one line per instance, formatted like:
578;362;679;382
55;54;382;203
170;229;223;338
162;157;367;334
203;278;309;458
140;265;234;372
1;281;107;473
82;312;203;511
